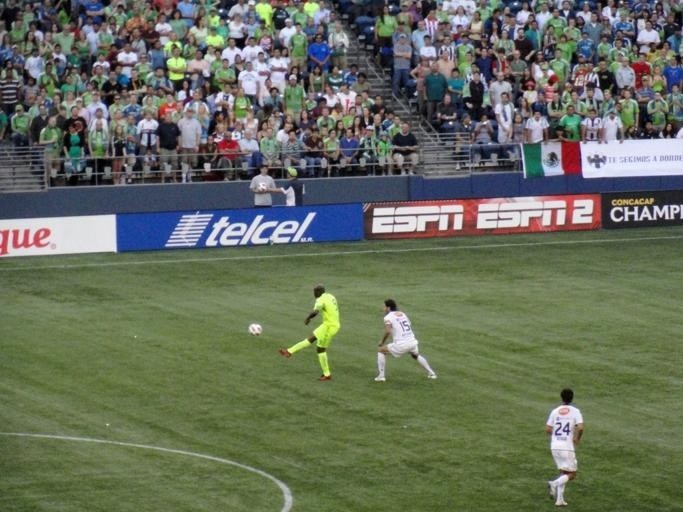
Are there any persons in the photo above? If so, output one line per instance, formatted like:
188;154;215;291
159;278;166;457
332;1;682;171
373;300;438;382
545;388;585;507
1;1;421;185
263;167;306;206
279;284;341;381
250;163;276;207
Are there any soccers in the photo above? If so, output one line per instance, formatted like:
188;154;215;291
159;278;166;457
248;323;262;336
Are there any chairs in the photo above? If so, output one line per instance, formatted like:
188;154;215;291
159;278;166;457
355;17;514;166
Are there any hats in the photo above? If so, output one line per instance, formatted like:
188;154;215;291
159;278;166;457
604;89;616;113
578;53;585;57
93;2;124;24
398;3;408;38
481;33;488;38
76;90;103;111
541;64;548;70
210;7;217;30
246;105;258;114
539;89;545;94
461;113;467;121
366;126;374;130
287;167;298;177
582;29;588;34
12;42;78;50
289;74;297;80
526;81;534;86
249;0;255;5
642;75;648;81
15;105;23;111
187;108;193;112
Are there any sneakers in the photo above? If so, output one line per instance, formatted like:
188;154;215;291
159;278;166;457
319;374;331;380
427;374;437;379
401;169;413;175
374;375;386;382
278;347;291;357
547;480;556;499
554;500;567;506
455;163;460;170
